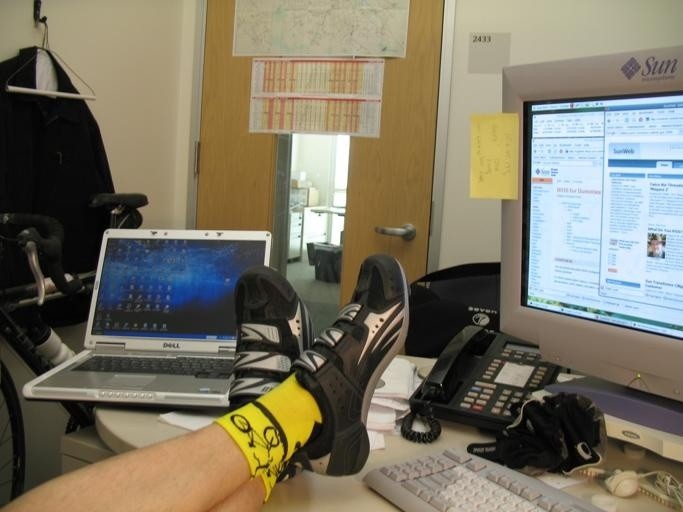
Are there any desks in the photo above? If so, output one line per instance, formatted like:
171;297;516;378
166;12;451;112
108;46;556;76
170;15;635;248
60;351;683;512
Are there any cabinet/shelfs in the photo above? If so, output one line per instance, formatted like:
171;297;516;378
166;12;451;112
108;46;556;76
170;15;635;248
288;206;329;264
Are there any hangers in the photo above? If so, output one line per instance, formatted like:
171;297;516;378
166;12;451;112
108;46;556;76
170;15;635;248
5;18;101;101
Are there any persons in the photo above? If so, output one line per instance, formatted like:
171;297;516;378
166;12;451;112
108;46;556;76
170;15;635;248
1;252;412;511
646;234;667;259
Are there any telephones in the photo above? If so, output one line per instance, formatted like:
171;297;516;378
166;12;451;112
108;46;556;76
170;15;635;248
408;326;562;433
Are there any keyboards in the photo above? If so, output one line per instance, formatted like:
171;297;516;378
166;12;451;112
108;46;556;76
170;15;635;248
362;445;608;512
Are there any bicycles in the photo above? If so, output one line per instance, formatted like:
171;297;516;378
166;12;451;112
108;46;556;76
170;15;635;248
0;193;148;505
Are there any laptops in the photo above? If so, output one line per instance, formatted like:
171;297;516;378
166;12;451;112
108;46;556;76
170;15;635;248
21;227;273;408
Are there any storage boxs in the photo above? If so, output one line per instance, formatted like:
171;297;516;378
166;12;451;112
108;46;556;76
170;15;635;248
291;178;320;206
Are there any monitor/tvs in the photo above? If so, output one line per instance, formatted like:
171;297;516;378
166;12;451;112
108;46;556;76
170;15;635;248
498;45;683;437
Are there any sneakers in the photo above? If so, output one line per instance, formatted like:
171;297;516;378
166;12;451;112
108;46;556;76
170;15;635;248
291;255;408;476
228;265;314;482
